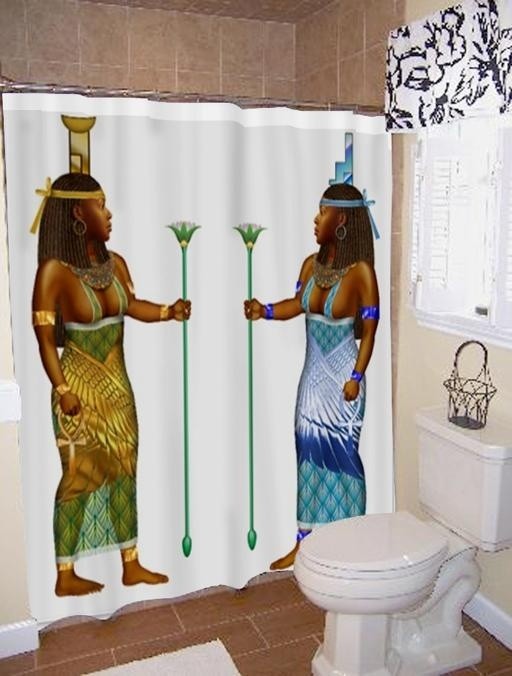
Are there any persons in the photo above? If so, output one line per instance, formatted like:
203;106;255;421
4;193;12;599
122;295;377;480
32;172;192;598
243;182;380;569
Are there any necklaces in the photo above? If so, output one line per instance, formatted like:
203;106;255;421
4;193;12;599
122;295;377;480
311;253;357;290
59;251;115;292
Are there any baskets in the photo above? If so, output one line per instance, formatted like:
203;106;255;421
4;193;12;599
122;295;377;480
443;340;496;428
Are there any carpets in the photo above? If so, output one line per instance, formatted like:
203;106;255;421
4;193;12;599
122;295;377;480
81;638;243;676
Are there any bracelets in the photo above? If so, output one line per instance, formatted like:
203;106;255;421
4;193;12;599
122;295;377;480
53;383;71;402
264;303;274;320
350;370;362;382
159;303;169;321
